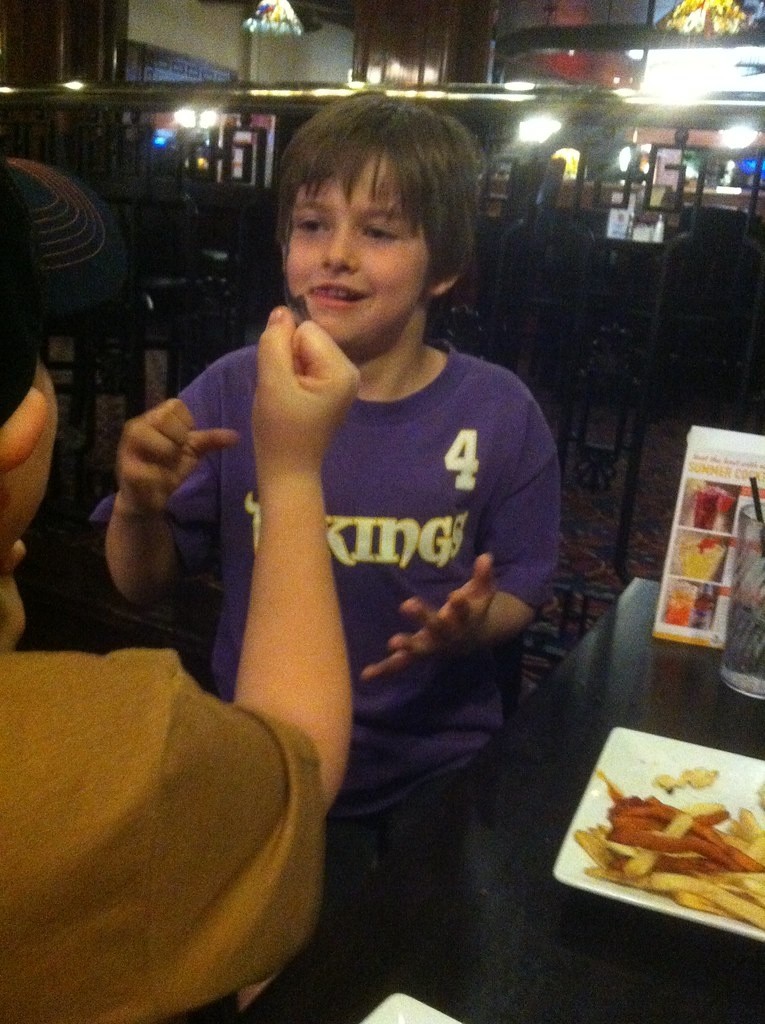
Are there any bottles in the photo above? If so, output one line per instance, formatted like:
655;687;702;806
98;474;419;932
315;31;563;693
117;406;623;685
691;584;715;630
652;212;666;244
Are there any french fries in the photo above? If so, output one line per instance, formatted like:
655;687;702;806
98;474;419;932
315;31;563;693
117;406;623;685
575;772;765;931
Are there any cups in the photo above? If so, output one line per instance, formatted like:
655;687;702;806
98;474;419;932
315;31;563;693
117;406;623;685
693;488;721;530
680;542;724;582
718;505;765;697
663;584;697;627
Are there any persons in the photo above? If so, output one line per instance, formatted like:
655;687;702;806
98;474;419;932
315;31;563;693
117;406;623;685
0;152;363;1024
88;92;561;1024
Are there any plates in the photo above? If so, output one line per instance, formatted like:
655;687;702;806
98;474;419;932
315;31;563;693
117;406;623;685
553;727;763;938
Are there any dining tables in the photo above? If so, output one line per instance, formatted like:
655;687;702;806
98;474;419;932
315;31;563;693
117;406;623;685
234;576;765;1023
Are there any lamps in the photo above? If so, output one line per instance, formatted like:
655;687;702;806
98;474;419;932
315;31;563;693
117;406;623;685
243;0;303;36
655;0;761;35
